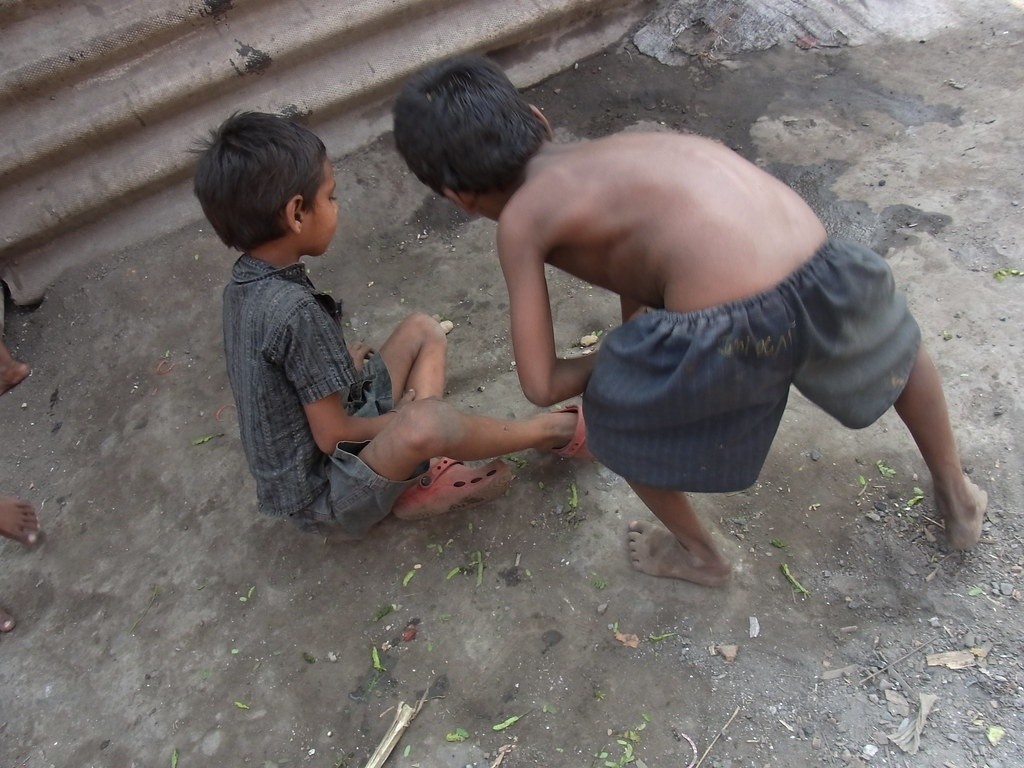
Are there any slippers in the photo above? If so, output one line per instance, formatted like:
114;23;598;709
392;456;517;521
548;404;597;461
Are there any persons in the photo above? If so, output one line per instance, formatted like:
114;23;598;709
0;495;41;632
392;45;990;588
0;339;32;397
181;109;596;542
